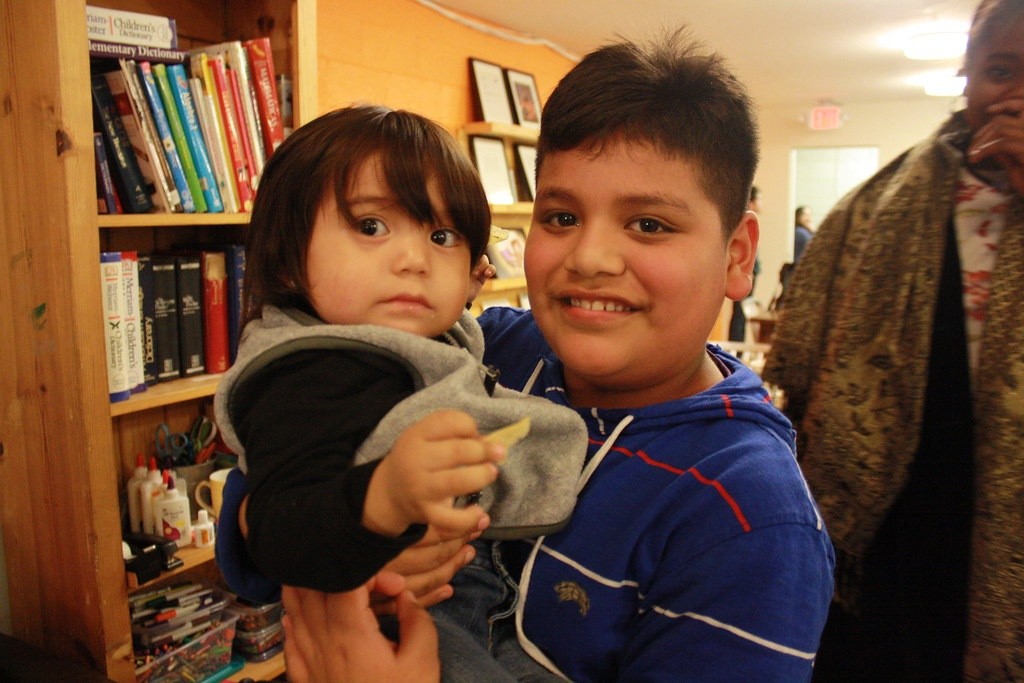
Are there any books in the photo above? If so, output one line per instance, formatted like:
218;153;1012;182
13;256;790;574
87;0;292;214
97;244;254;403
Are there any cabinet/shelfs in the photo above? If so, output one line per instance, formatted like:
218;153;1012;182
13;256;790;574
464;121;534;293
0;0;316;683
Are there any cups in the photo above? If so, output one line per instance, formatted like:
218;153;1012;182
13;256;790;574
175;462;214;520
196;468;234;517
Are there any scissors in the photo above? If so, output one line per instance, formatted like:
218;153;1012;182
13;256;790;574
154;414;217;466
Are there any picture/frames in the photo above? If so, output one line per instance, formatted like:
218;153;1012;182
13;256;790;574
468;57;514;124
468;134;514;205
513;142;536;202
485;228;526;279
503;67;542;128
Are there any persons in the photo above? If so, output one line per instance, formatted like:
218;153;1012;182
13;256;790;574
729;186;760;358
760;4;1024;683
796;205;817;264
213;40;839;683
214;103;589;682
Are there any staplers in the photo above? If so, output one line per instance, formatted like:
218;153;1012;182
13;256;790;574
123;531;184;572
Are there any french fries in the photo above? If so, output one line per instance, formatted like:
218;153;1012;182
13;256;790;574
481;415;531;448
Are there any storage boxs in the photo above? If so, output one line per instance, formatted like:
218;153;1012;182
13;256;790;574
135;591;235;648
234;623;281;654
222;601;282;633
135;607;240;683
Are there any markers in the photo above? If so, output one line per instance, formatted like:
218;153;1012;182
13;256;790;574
133;580;219;649
